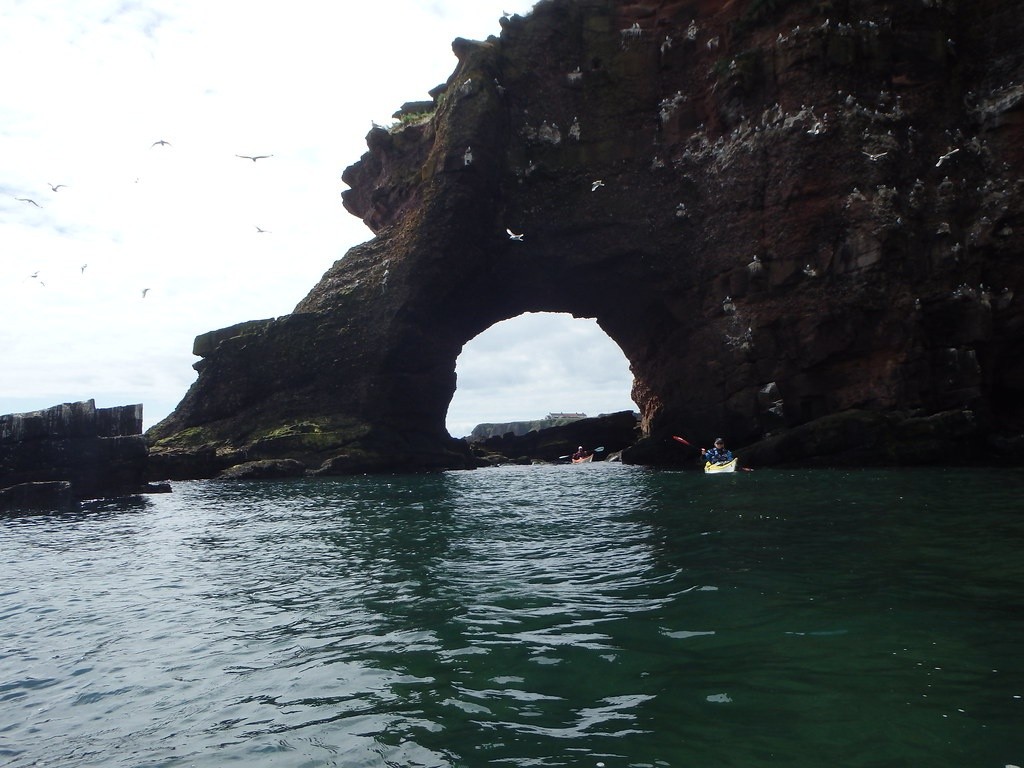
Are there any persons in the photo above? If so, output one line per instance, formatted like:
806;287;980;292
701;438;734;465
572;446;587;460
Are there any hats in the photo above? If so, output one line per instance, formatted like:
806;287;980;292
714;438;724;443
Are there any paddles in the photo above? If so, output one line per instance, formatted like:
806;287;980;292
673;435;752;473
559;447;604;459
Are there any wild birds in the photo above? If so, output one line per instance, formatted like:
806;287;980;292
592;179;605;192
140;287;150;298
14;182;67;208
81;262;89;273
936;149;960;167
506;228;525;242
254;225;272;233
862;152;887;161
234;153;274;162
149;140;172;148
29;269;45;287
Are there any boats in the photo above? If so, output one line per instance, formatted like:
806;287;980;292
704;457;738;473
570;454;595;464
